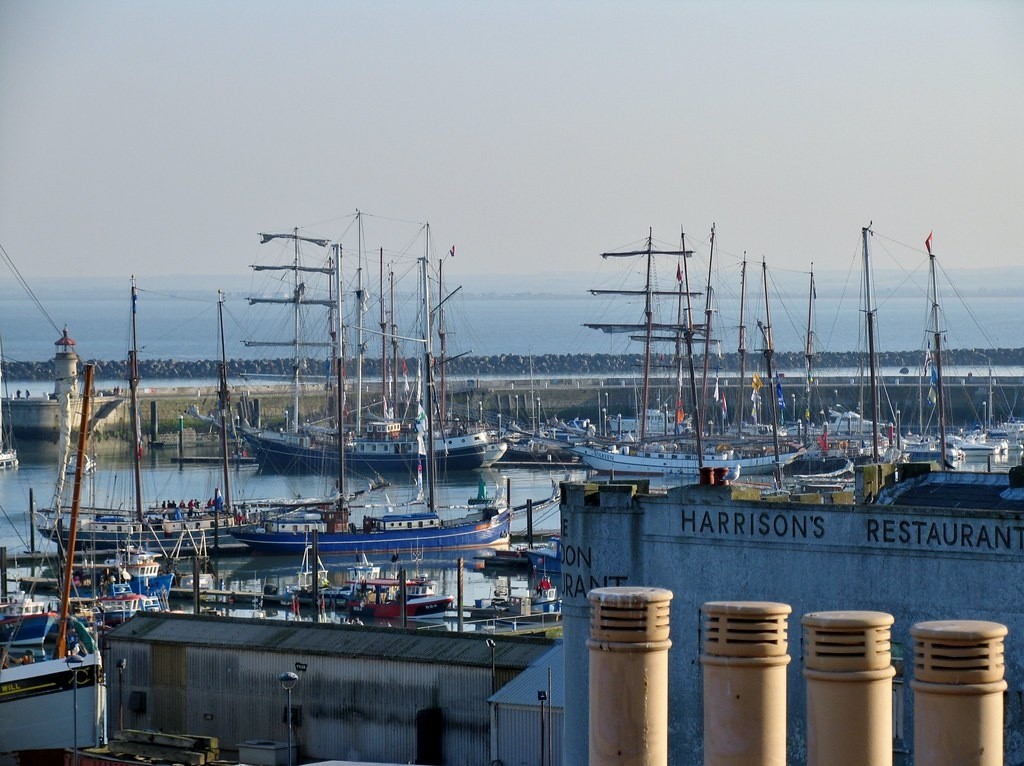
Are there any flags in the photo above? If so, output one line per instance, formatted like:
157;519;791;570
659;353;664;360
136;400;144;461
675;369;831;452
925;233;933;253
677;264;682;281
715;341;722;359
229;405;237;439
813;279;817;299
923;341;939;407
215;488;223;513
329;355;430;502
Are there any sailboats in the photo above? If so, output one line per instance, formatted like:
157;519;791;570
0;373;19;469
184;208;545;469
0;241;175;752
559;222;1024;502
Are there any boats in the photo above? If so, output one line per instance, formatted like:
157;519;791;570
20;512;216;606
67;451;96;474
264;527;456;620
475;552;561;617
520;530;561;573
548;418;597;442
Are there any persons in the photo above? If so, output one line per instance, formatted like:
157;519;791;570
16;389;21;399
114;386;122;396
162;499;201;517
25;389;30;399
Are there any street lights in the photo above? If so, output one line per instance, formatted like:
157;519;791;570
279;672;298;766
65;655;83;766
